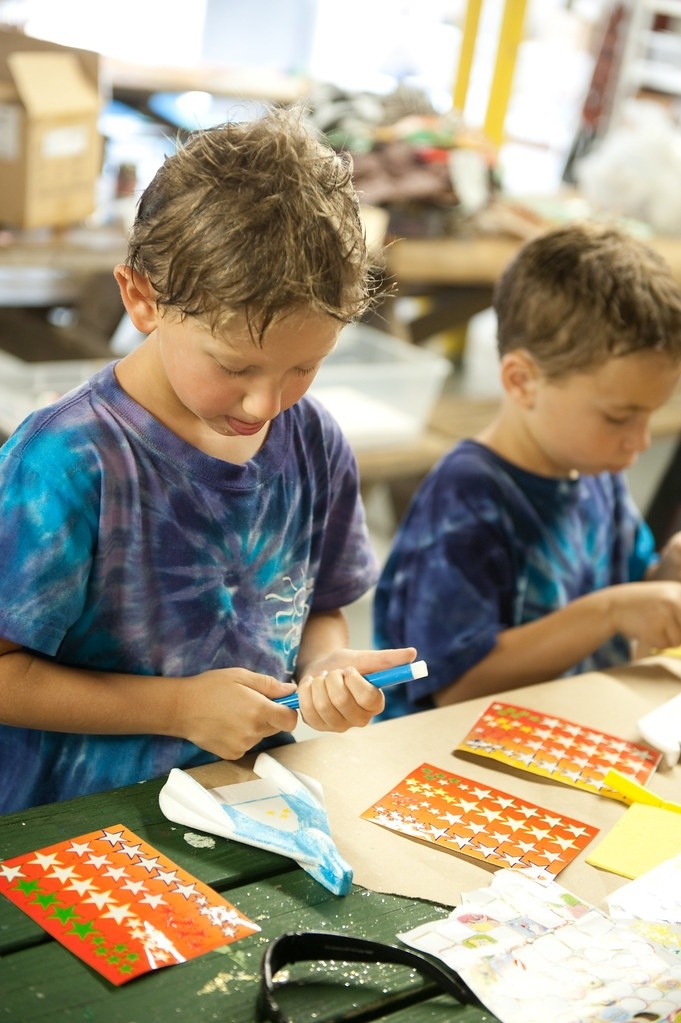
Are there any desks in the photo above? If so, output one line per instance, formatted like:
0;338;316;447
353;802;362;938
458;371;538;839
0;352;514;495
0;637;681;1022
360;234;534;346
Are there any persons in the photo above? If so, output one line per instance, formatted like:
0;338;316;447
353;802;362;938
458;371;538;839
372;219;681;725
0;102;418;822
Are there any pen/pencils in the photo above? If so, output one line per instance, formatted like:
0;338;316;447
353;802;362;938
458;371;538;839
272;658;430;719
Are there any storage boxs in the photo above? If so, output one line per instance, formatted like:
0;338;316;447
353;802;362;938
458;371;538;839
4;23;109;230
297;325;453;455
